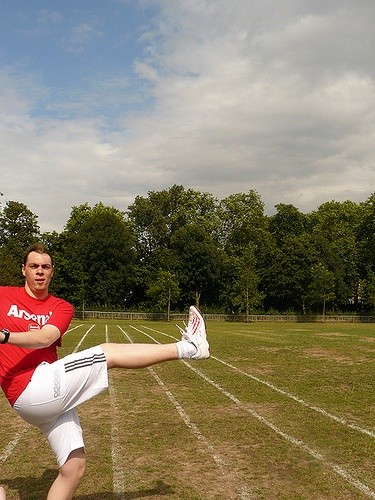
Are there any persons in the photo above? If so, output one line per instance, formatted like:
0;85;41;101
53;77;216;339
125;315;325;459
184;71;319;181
0;243;210;500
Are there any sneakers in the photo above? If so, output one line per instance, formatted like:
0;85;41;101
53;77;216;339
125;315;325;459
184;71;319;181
176;305;211;360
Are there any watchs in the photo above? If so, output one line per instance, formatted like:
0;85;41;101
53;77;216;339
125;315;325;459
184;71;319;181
0;328;10;344
